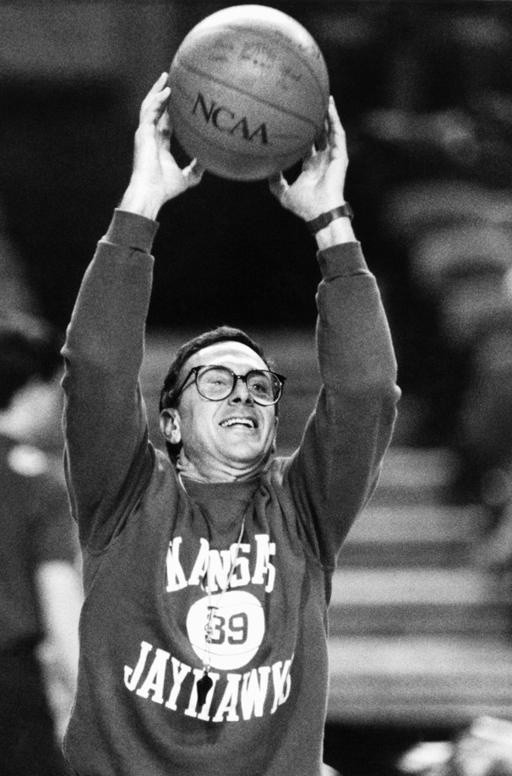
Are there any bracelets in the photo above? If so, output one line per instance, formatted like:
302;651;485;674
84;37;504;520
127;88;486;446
304;201;355;234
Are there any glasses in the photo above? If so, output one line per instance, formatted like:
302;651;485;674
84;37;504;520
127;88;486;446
174;365;287;406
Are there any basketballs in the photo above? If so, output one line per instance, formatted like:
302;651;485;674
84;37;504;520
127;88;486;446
167;4;329;181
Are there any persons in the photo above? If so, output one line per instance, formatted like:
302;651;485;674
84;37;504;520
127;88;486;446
1;347;85;774
59;70;403;774
